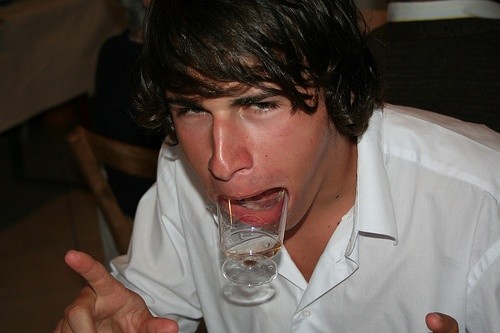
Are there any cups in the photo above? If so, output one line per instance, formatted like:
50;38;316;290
216;186;289;288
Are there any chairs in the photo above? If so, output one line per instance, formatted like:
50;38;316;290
67;126;157;256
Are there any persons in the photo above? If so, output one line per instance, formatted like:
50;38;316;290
53;1;500;333
